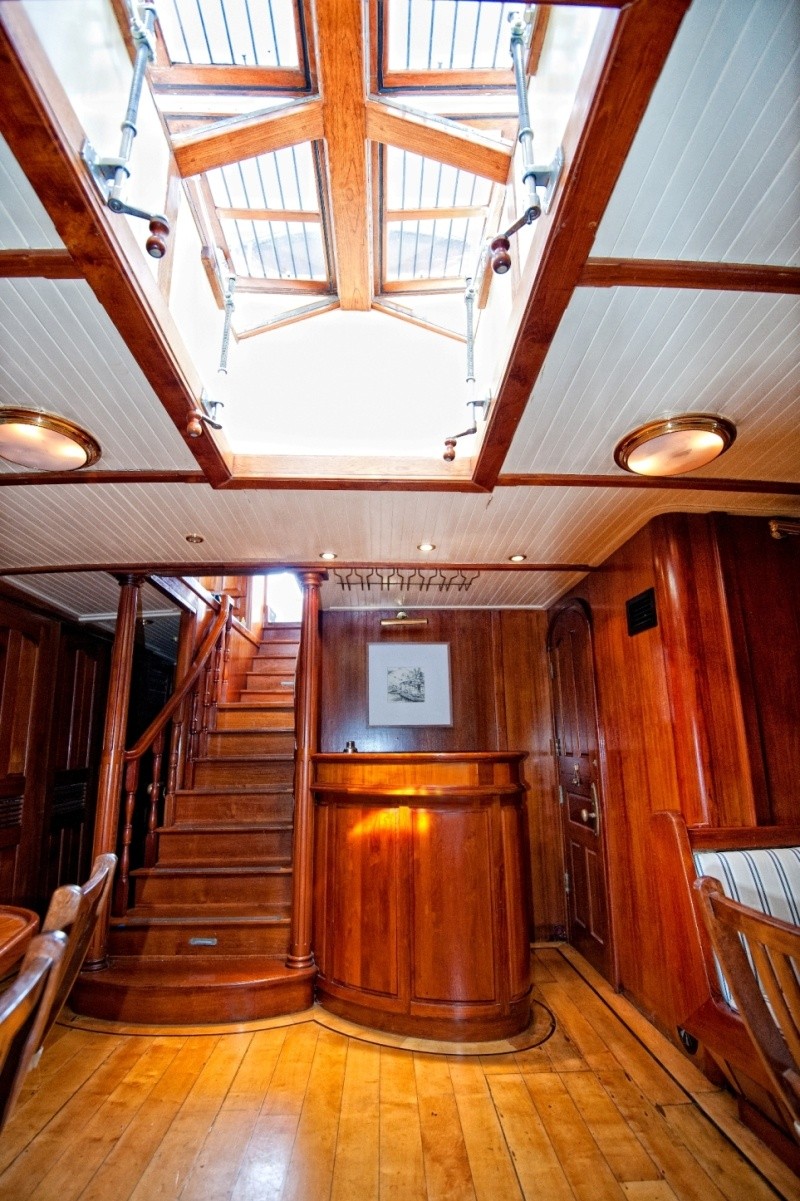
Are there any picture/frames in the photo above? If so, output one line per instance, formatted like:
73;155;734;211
366;642;454;727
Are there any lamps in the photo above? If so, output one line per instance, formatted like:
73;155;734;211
0;405;102;470
613;413;738;477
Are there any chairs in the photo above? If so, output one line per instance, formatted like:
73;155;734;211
0;851;119;1124
650;810;800;1181
693;876;800;1138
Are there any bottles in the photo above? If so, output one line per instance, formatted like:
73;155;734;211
343;741;358;753
